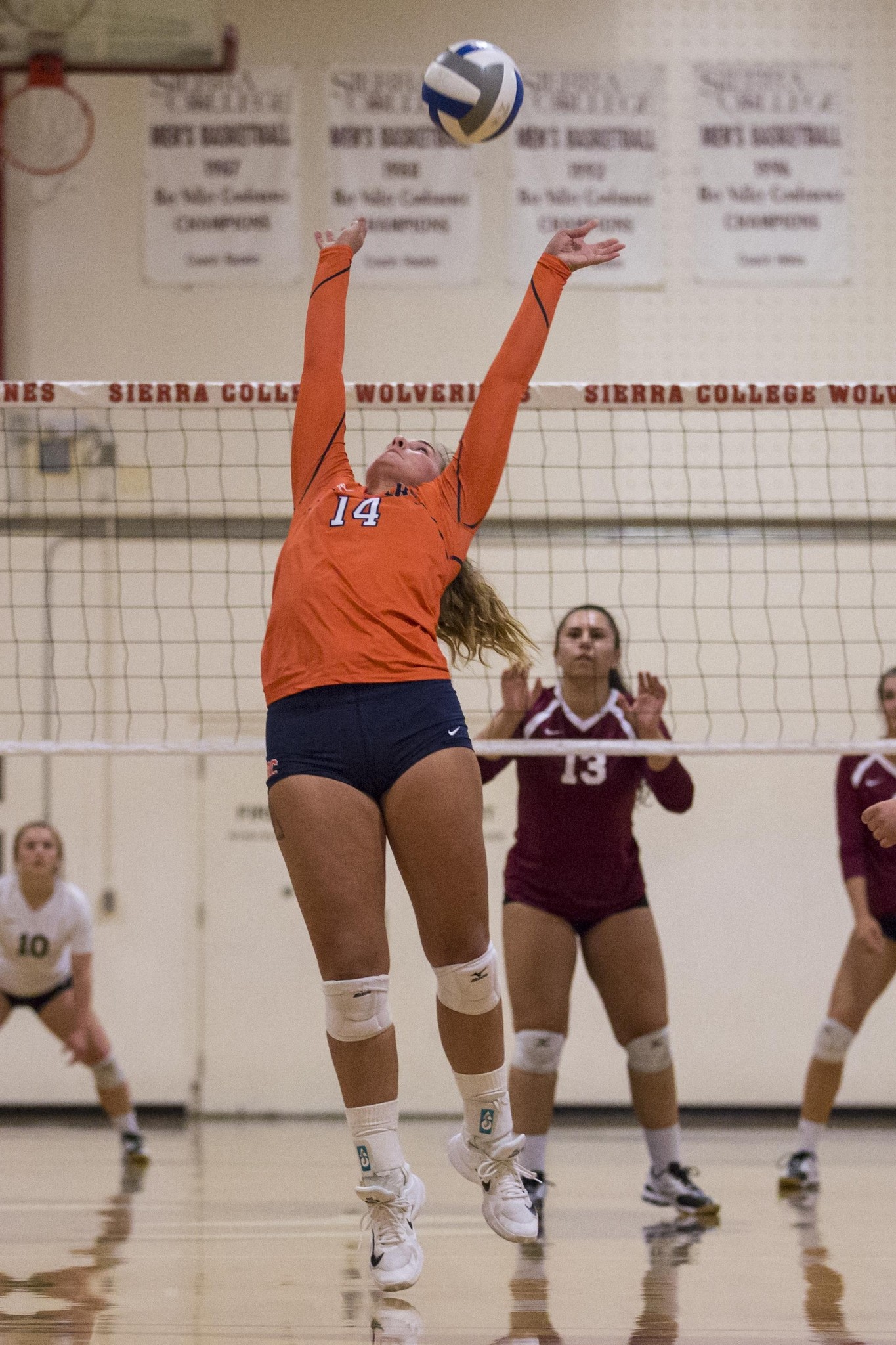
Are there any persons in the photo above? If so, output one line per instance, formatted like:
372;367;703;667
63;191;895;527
471;603;722;1239
0;821;153;1164
776;666;896;1188
262;216;625;1293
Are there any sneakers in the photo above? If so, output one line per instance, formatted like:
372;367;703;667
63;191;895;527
447;1123;538;1242
522;1170;548;1235
355;1172;426;1291
644;1158;718;1215
777;1145;822;1188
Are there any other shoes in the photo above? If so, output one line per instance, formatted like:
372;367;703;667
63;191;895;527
123;1132;148;1160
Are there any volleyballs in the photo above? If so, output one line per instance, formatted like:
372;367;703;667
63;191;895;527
421;40;525;146
